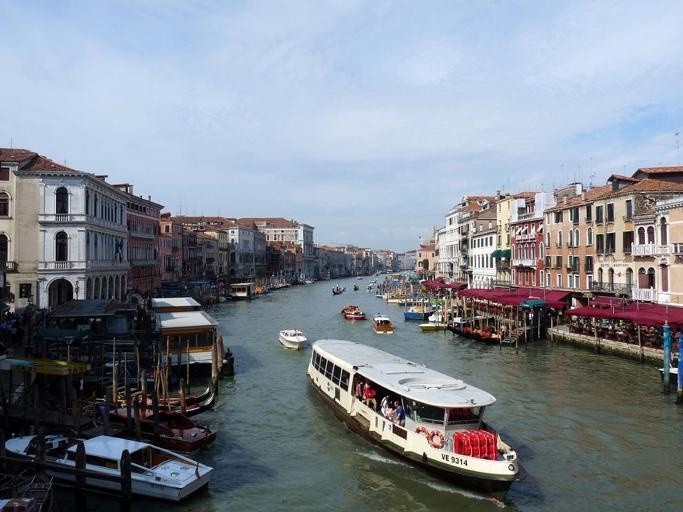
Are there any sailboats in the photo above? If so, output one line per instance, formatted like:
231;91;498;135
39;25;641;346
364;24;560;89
207;271;315;302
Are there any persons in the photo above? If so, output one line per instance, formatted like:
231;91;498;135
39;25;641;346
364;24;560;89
355;379;364;403
411;401;424;417
379;393;393;420
389;400;405;428
362;381;377;412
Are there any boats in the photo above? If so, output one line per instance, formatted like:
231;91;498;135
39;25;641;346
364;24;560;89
1;431;216;506
304;336;527;501
1;284;232;460
276;328;308;351
332;266;518;344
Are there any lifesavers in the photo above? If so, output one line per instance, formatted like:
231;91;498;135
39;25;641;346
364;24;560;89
426;430;445;448
415;427;430;440
